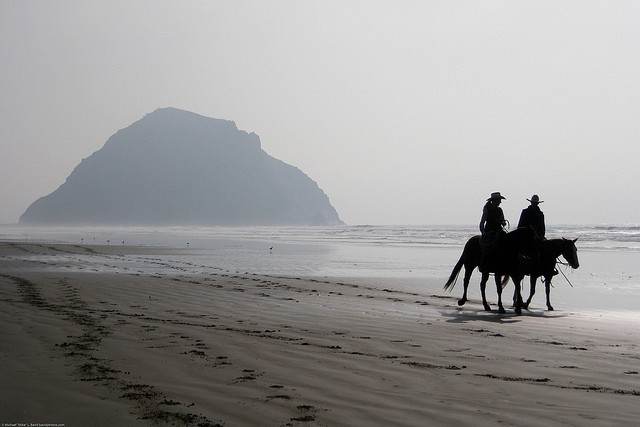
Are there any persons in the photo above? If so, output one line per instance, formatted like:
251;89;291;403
517;195;546;241
480;192;506;271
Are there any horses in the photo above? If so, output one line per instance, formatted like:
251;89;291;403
443;225;541;314
502;237;579;311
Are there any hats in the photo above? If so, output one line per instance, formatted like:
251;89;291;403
486;192;506;201
526;195;544;203
